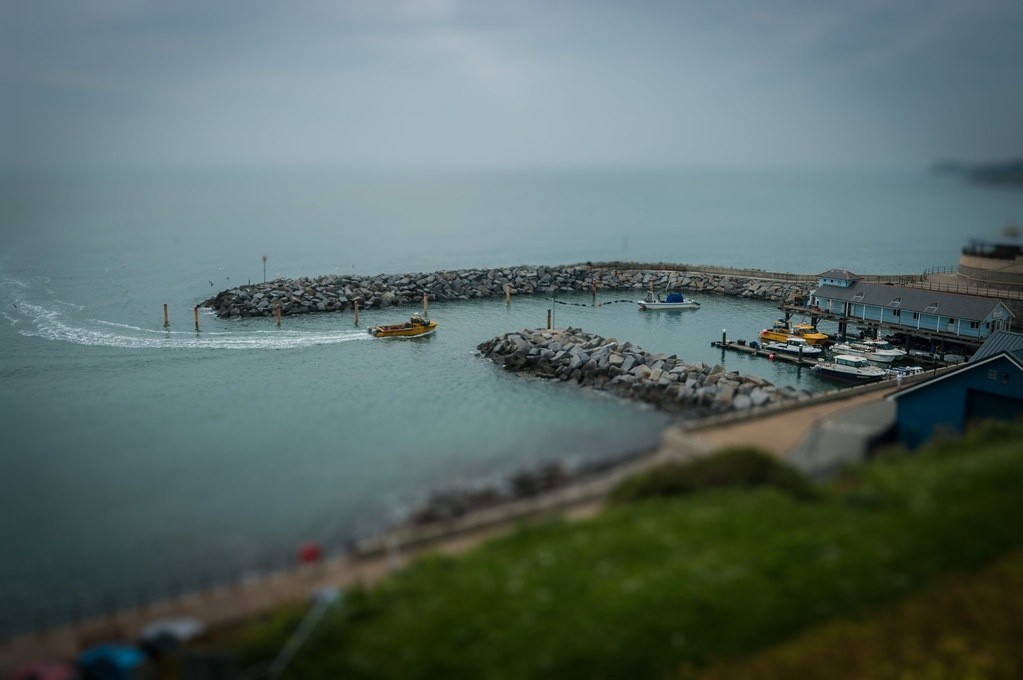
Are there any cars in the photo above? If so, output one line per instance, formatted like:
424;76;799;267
7;616;205;680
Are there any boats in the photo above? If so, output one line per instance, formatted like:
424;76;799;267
761;336;822;358
758;318;829;348
809;354;887;385
637;290;701;310
827;336;907;364
366;312;438;338
880;365;924;379
